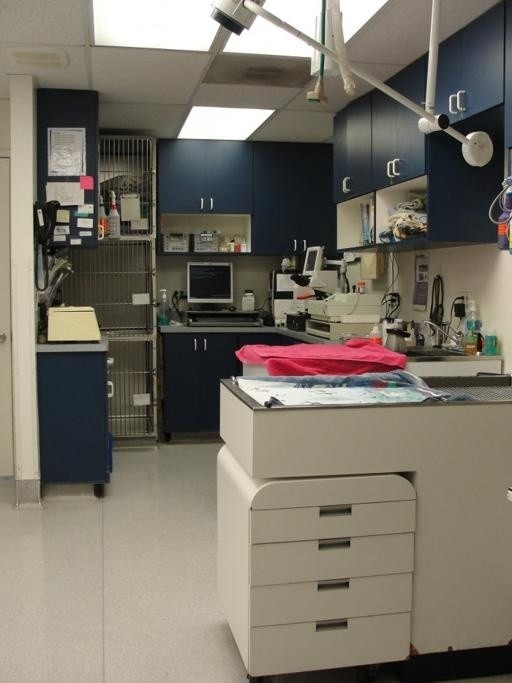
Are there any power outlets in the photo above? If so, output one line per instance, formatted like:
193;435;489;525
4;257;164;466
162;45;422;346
461;289;475;312
172;288;184;300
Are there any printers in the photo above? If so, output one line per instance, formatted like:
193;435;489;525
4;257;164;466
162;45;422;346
305;291;390;339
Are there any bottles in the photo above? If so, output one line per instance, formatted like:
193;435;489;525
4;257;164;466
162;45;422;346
369;321;382;346
357;281;365;293
225;233;247;252
484;328;496;353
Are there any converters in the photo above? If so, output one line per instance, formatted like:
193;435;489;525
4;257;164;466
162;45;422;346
454;303;465;316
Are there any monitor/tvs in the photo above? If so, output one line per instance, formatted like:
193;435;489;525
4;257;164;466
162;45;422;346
187;261;234;310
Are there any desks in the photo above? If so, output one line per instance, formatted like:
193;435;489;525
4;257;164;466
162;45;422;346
37;332;113;501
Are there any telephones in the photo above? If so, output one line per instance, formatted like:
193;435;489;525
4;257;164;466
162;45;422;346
34;200;60;245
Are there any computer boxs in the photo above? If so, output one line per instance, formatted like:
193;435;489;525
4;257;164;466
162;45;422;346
268;269;338;327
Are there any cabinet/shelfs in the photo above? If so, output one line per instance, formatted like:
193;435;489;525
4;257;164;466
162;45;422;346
430;2;503;128
214;439;416;682
334;91;374;252
156;134;251;255
168;328;239;444
376;52;499;252
36;87;101;249
250;139;336;256
